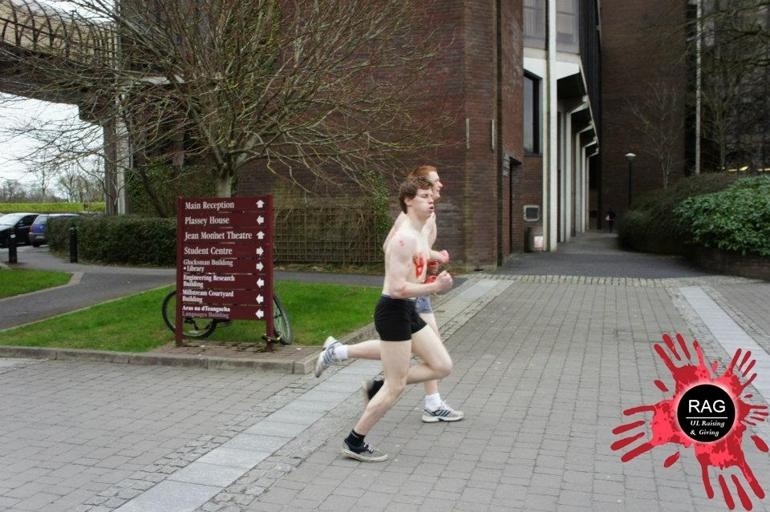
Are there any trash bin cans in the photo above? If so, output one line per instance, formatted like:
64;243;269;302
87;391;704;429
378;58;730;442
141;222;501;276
528;226;543;251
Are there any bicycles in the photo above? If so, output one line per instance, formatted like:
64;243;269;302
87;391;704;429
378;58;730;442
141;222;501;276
161;289;293;346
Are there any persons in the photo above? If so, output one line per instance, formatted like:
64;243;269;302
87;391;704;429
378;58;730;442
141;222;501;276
606;207;618;234
339;177;456;464
314;163;468;425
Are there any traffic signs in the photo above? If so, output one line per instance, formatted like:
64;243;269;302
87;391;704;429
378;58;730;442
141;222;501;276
177;195;275;352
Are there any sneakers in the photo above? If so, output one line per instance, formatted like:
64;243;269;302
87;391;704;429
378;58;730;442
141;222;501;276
422;400;464;423
341;438;388;462
360;378;377;401
314;336;342;378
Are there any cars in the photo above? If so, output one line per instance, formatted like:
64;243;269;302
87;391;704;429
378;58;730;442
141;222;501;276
0;212;40;248
28;213;80;247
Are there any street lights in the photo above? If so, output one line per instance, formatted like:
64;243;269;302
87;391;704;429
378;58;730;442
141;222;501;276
624;151;636;208
